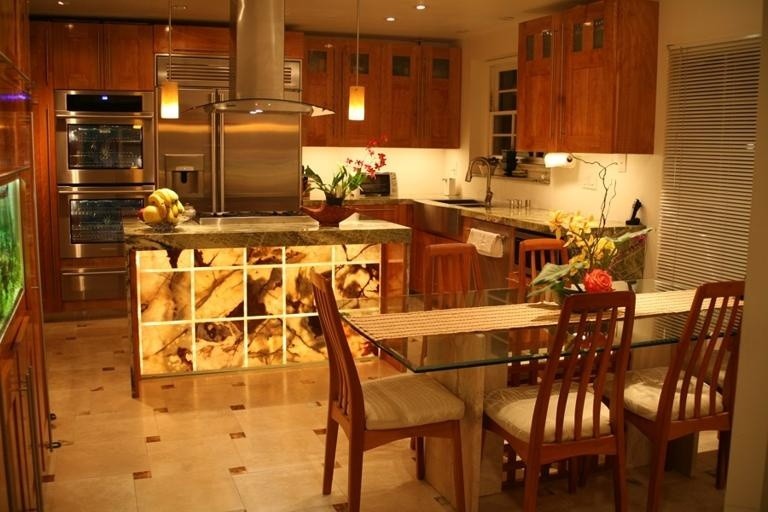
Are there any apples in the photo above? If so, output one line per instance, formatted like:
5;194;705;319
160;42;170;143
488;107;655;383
137;205;162;224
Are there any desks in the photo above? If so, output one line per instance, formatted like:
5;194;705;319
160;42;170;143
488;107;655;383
335;282;747;510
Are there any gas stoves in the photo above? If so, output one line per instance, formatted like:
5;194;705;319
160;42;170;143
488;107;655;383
198;208;312;225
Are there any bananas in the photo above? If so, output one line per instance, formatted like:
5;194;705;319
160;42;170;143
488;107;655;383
147;187;185;219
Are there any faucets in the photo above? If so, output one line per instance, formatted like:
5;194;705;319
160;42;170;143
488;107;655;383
465;157;490;193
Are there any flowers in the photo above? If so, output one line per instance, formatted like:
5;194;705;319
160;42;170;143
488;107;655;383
302;136;387;197
527;212;653;296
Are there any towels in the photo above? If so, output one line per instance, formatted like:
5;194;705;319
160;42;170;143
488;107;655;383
467;228;503;258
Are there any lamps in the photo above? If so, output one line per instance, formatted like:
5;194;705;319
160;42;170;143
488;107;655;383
347;0;366;120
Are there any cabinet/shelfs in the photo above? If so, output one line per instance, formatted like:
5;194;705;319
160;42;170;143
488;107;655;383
347;205;413;228
515;0;660;155
379;39;462;148
44;22;236;90
302;34;380;146
0;0;58;511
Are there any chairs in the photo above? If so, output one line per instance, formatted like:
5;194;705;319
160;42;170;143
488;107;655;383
517;238;576;287
309;266;468;511
483;287;636;510
592;280;747;510
421;241;487;293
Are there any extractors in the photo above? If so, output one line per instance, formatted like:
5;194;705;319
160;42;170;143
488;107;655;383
184;1;336;117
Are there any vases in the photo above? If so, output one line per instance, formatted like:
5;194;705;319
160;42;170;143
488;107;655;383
325;194;343;206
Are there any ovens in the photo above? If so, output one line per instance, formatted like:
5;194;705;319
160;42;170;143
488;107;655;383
51;89;159;302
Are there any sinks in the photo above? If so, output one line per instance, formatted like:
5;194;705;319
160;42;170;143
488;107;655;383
459;204;485;209
431;200;482;203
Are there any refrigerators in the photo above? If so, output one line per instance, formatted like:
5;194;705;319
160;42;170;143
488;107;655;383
154;88;303;212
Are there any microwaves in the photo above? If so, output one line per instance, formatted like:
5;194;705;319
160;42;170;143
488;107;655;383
353;171;399;200
